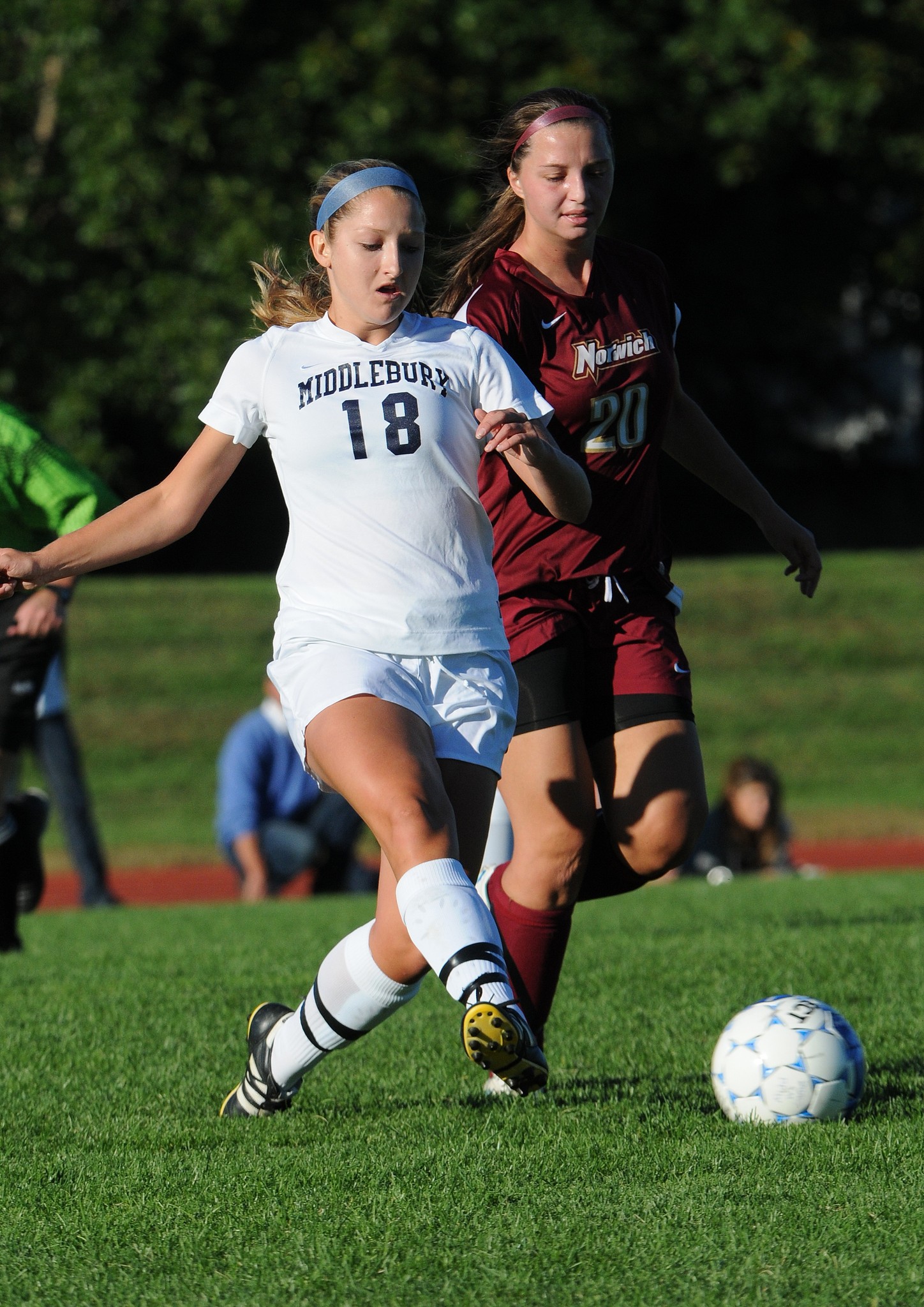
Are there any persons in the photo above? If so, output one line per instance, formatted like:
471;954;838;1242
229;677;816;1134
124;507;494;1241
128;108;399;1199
417;86;824;1099
2;399;796;952
0;156;594;1118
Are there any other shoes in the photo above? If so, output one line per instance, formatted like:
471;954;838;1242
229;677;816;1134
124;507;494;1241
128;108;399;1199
482;1072;520;1099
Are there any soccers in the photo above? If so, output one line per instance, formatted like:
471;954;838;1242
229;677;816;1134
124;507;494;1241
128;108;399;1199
710;993;864;1125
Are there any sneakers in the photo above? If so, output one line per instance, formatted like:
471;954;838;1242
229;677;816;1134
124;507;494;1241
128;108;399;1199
460;1002;548;1098
219;1003;303;1117
